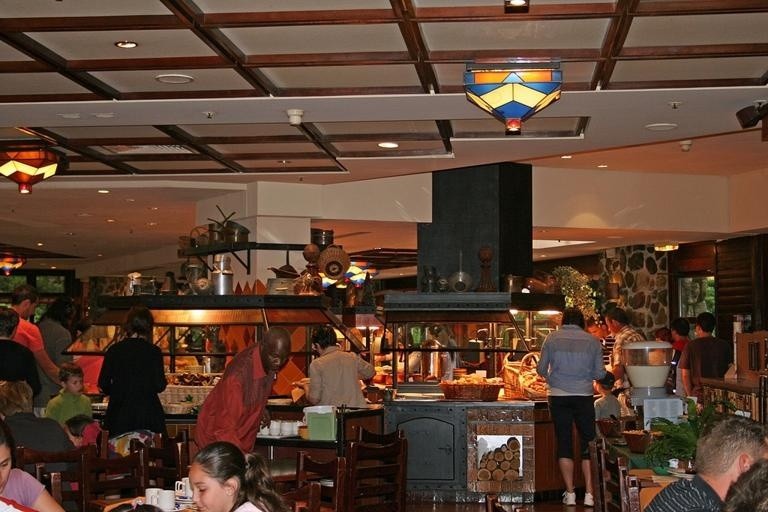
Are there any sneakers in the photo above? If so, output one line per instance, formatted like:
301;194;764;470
562;491;576;505
584;493;594;506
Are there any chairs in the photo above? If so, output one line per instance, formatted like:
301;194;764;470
0;360;766;511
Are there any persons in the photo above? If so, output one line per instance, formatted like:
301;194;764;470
643;412;768;512
295;326;376;411
192;326;291;453
538;307;606;507
1;284;169;512
189;442;288;512
586;307;732;438
349;324;458;380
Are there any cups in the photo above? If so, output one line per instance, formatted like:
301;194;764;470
145;477;193;512
685;456;696;472
669;458;678;468
623;341;684;429
99;337;111;351
259;420;301;436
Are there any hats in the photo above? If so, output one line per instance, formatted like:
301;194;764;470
597;371;615;385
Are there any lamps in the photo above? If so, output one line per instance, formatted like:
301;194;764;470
0;143;70;197
0;248;30;280
459;60;566;137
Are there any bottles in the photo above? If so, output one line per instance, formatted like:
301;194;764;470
421;265;437;292
201;356;210;374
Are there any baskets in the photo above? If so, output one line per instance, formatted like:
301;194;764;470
439;352;548;402
317;244;351;280
158;386;215;415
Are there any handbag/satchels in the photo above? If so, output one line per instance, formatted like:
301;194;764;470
665;365;676;394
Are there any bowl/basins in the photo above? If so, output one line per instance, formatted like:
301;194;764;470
594;419;623;435
311;226;334;245
298;424;308;439
621;432;663;453
380;388;397;400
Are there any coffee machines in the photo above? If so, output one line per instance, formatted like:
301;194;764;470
448;271;473;294
